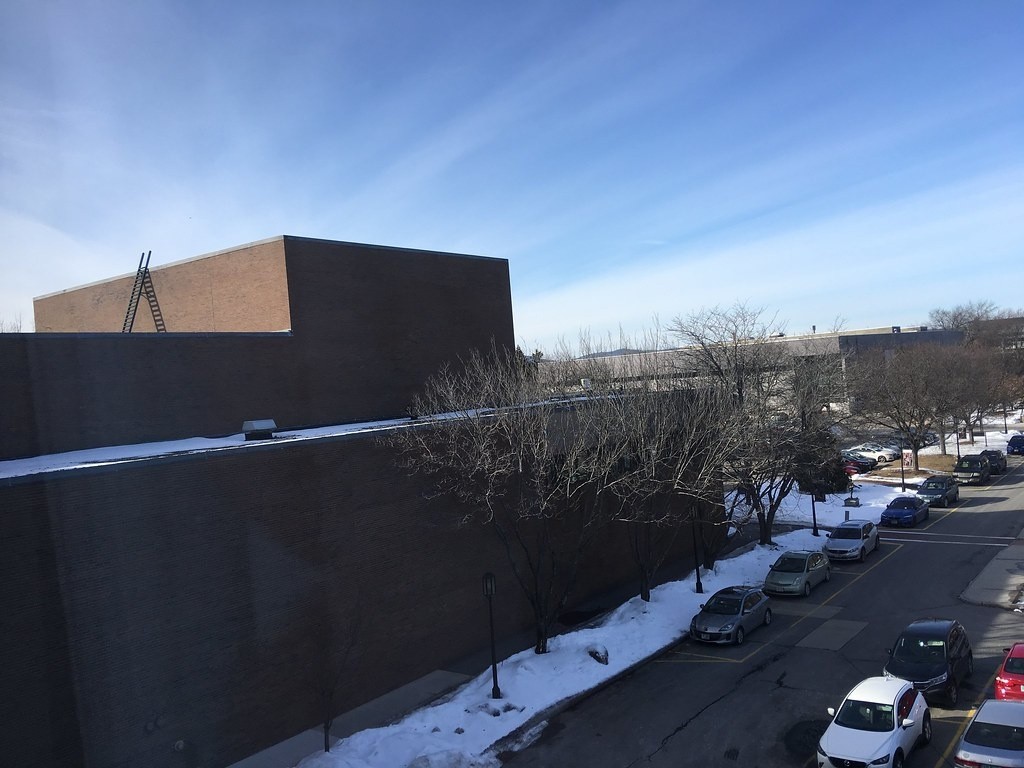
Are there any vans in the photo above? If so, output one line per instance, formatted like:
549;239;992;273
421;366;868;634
822;519;880;564
951;454;992;486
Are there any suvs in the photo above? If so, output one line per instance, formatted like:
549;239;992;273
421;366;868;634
980;449;1008;475
882;615;975;709
817;675;934;767
915;474;960;508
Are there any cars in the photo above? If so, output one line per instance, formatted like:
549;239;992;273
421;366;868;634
689;585;773;646
842;450;878;469
994;640;1024;702
887;441;901;458
881;496;930;527
840;453;870;472
871;426;939;447
1006;434;1024;455
765;548;832;597
953;698;1024;768
771;412;788;421
819;459;862;477
827;455;870;473
847;446;894;463
851;442;897;457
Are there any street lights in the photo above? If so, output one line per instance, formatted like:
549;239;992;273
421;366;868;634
481;571;502;699
